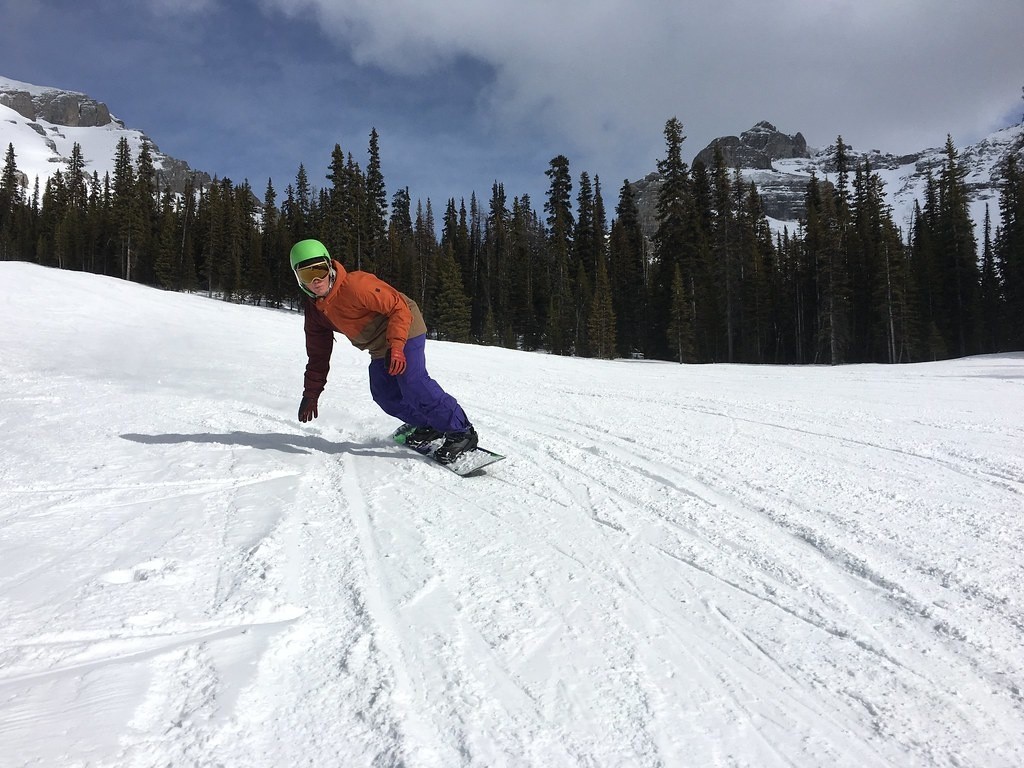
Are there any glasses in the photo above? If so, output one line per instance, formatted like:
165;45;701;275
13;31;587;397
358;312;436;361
295;258;331;285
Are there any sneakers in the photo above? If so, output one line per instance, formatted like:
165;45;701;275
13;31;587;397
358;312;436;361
406;423;442;446
435;426;479;464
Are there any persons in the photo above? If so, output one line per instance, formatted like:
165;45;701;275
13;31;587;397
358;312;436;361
287;238;476;465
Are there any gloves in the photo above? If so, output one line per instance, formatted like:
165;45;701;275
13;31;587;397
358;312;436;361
384;346;407;376
299;396;318;422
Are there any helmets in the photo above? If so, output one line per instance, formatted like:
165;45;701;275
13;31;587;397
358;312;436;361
289;239;332;289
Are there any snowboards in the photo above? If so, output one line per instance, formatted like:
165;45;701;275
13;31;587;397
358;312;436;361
392;423;506;477
